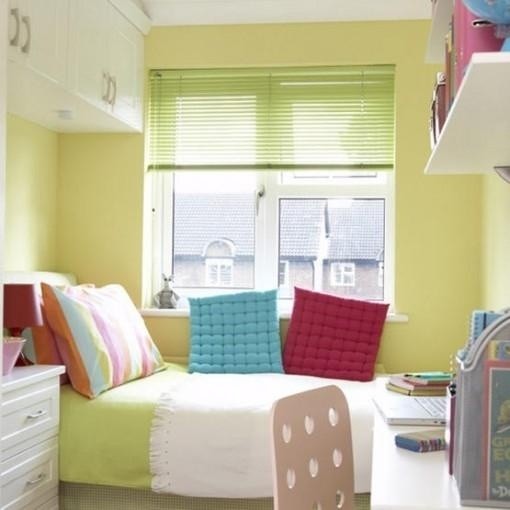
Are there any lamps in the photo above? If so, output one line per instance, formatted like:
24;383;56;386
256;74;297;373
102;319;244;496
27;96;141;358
3;279;44;365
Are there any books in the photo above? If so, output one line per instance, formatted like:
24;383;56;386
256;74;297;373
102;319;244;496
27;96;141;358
386;372;456;452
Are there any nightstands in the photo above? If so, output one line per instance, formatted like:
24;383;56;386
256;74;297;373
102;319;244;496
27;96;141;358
1;363;67;509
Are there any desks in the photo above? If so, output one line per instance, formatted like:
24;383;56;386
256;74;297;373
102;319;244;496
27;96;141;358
371;368;508;509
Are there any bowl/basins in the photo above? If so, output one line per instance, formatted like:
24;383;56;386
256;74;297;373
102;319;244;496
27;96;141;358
2;336;27;375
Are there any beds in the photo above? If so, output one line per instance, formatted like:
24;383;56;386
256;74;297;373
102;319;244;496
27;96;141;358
1;269;390;509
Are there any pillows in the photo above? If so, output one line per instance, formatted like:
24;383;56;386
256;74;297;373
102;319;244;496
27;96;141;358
39;282;169;400
281;286;392;382
186;288;286;376
16;281;69;385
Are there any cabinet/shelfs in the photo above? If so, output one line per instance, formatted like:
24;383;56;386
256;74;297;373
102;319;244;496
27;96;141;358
423;1;510;178
7;0;151;135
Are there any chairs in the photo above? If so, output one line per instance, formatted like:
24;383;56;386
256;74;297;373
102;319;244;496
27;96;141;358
269;385;356;510
